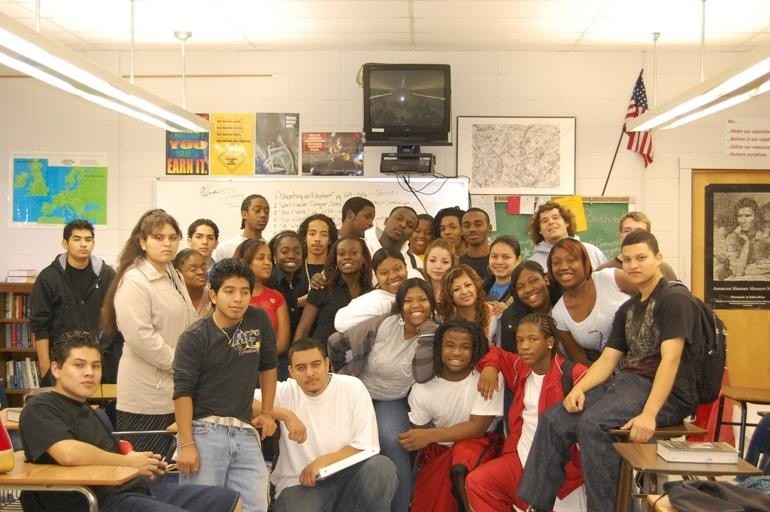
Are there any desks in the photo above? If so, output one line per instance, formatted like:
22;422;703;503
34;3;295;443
0;381;139;510
609;379;770;512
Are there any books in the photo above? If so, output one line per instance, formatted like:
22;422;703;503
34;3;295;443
6;275;37;283
3;290;40;388
8;267;38;276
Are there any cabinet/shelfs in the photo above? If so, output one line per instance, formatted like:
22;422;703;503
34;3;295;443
0;281;43;409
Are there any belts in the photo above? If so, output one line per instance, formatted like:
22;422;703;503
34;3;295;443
202;415;261;447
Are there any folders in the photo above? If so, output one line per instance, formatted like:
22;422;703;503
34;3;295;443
316;446;380;477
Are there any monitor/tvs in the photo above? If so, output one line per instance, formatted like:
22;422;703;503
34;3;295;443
364;63;451;141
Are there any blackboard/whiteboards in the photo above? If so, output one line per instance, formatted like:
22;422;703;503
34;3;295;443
493;201;629;262
149;177;469;264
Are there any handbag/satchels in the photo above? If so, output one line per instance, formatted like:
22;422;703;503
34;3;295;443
663;480;769;512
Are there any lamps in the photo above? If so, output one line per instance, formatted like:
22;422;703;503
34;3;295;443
625;0;770;136
0;0;215;138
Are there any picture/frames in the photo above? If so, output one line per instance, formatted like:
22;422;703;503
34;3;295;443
454;115;578;197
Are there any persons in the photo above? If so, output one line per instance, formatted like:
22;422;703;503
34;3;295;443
97;206;198;471
29;218;117;392
174;194;696;511
16;326;249;511
727;197;770;277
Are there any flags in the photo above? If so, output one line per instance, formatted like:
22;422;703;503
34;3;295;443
623;76;652;168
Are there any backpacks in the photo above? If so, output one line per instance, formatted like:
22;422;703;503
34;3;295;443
621;281;727;403
